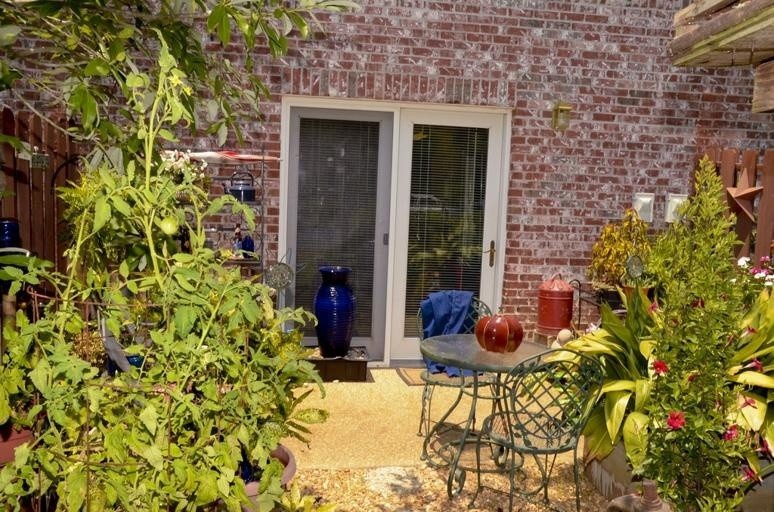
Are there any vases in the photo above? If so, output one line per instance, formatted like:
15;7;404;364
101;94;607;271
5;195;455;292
310;266;356;358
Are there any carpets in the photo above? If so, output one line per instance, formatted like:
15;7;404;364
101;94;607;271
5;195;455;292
397;366;499;387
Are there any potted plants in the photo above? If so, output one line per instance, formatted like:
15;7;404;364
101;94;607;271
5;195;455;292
590;210;656;303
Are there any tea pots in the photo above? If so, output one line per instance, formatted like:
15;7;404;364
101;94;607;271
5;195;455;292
221;169;256;201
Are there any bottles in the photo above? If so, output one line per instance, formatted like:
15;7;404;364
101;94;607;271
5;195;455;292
231;222;243;256
241;234;256;259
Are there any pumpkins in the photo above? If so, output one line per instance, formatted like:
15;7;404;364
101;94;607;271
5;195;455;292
475;313;523;354
